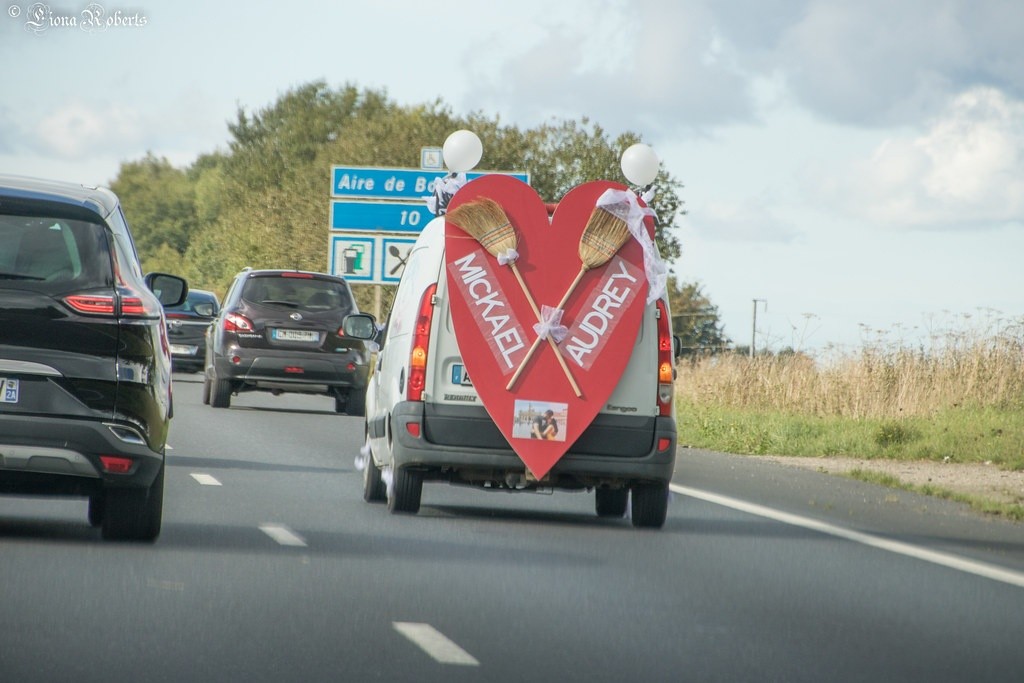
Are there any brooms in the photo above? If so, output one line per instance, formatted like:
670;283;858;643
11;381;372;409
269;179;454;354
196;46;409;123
505;187;647;391
441;195;582;397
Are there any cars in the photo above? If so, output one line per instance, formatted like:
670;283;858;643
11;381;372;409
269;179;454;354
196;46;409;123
149;285;218;376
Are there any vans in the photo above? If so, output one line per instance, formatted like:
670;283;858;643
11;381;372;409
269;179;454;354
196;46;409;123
342;199;681;530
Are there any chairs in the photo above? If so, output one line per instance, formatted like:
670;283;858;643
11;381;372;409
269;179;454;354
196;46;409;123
247;286;270;302
306;292;336;307
17;228;74;279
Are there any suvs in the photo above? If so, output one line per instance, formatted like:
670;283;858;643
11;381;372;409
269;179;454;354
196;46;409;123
192;266;375;418
2;170;191;542
